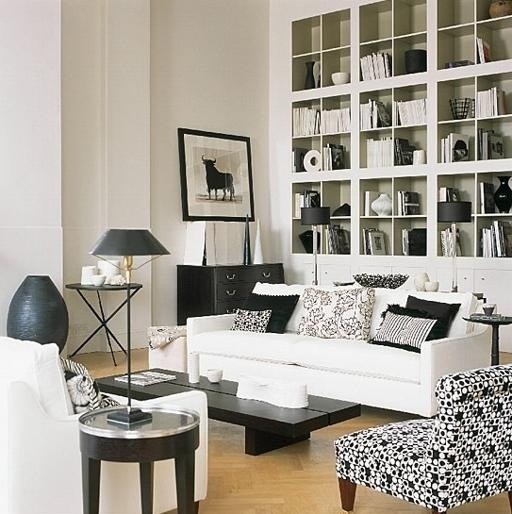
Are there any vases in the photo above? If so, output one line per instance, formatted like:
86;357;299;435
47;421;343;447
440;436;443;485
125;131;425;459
7;274;69;355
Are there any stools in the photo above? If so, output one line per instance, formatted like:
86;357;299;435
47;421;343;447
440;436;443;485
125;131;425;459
148;326;187;373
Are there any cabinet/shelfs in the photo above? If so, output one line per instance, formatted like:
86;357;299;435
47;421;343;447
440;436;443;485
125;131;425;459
435;1;511;70
292;8;351;93
292;179;351;255
176;263;286;326
298;263;351;285
358;175;428;255
359;2;428;84
436;71;511;164
437;171;512;258
358;84;428;170
360;265;424;282
292;94;353;173
455;257;511;353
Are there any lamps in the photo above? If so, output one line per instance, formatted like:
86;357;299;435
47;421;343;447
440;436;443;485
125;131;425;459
88;229;171;426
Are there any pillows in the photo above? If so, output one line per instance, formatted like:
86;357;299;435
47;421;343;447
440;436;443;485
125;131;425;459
229;282;461;354
0;337;125;416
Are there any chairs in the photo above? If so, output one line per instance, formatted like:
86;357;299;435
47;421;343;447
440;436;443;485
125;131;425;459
333;363;512;514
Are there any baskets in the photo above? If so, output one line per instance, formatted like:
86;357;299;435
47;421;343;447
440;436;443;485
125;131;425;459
448;97;471;118
352;274;409;288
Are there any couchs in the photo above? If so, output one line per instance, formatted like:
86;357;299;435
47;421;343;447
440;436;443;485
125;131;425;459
0;374;209;514
187;303;492;418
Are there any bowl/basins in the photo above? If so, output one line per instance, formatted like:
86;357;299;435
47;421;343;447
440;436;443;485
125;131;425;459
91;275;106;286
331;72;349;85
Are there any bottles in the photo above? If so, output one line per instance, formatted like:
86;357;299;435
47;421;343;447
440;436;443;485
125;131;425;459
302;61;316;89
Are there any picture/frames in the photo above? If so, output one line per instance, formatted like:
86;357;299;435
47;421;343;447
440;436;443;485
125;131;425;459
178;127;255;223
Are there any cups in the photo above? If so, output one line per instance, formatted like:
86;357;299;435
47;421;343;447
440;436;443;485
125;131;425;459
425;281;439;292
187;351;223;383
482;304;497;317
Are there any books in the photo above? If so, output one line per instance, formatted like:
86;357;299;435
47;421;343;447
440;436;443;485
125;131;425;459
294;191;319;218
477;218;510;257
396;187;423;216
291;146;310;172
475;86;508;118
116;369;177;388
363;188;382;216
318;224;351;254
476;182;497;212
360;97;430;131
324;142;349;170
359;51;392;81
438;130;472;164
476;125;503;160
434;223;471;260
292;104;352;135
440;185;461;200
366;135;415;168
361;228;386;256
477;36;492;64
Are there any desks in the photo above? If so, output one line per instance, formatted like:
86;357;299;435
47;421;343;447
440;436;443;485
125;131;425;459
463;314;511;366
66;283;143;367
79;405;201;514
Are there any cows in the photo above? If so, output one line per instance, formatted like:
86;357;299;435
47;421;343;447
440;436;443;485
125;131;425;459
201;155;235;201
